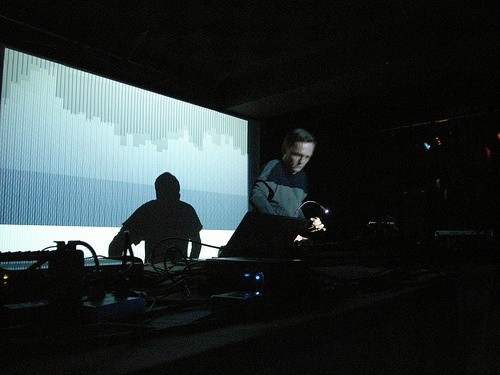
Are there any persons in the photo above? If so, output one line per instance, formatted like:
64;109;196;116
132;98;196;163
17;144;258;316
248;127;317;220
105;172;203;263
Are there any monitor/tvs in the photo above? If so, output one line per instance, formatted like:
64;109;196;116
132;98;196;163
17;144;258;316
219;212;312;258
